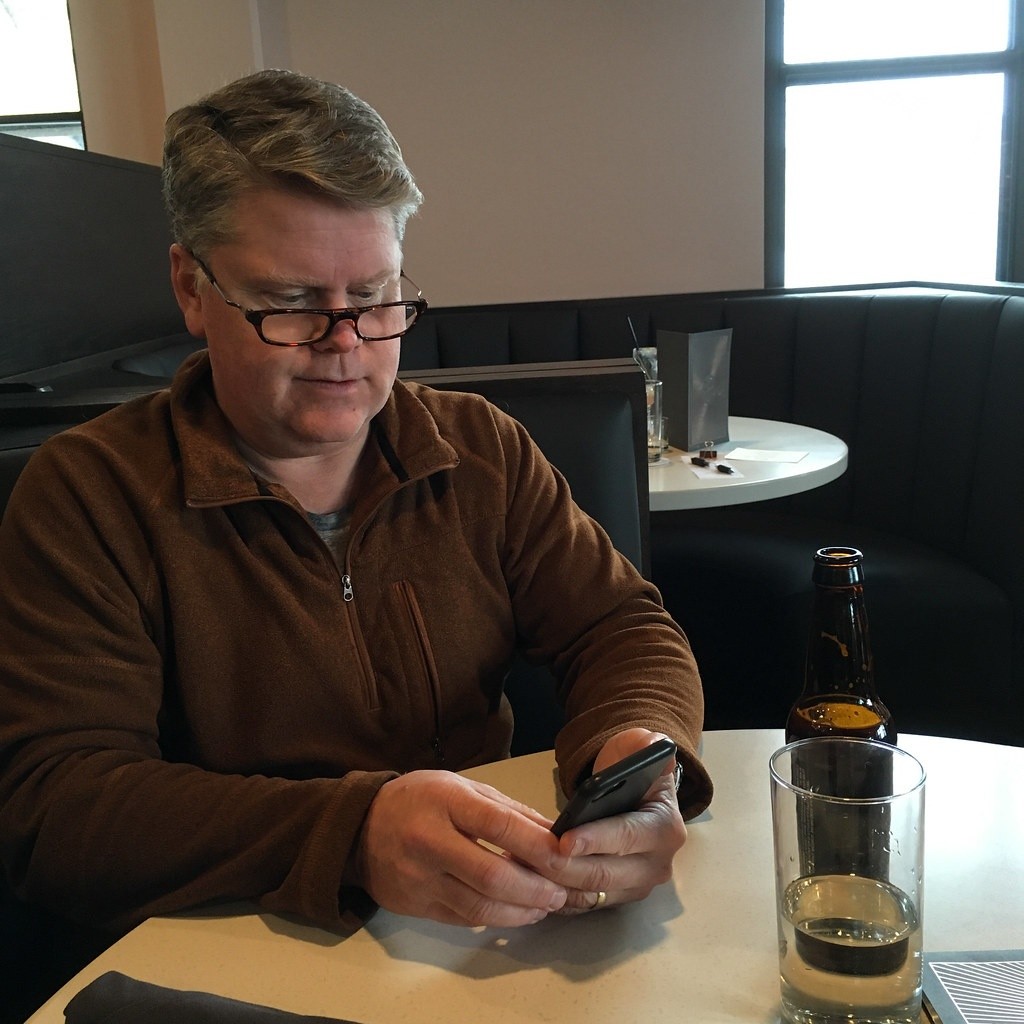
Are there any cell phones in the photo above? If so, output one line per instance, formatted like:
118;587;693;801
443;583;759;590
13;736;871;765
548;739;678;840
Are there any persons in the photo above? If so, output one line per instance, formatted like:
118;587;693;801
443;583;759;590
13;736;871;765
0;67;713;938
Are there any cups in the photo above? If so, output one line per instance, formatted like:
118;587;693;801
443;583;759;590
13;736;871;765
768;737;925;1023
645;380;665;463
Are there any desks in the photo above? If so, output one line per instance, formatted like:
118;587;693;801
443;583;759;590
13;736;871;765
21;727;1023;1024
648;416;848;511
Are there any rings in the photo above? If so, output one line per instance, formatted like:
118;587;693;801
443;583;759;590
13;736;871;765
588;891;606;912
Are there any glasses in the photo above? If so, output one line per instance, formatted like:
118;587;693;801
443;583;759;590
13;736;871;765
189;252;429;347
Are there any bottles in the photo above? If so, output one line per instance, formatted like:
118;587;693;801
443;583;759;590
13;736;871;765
783;548;909;973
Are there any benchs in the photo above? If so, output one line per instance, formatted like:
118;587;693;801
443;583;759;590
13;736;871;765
1;360;651;751
399;279;1024;744
